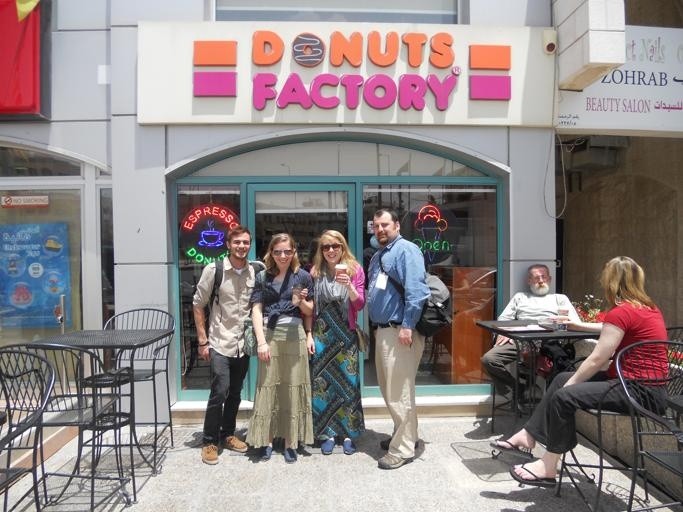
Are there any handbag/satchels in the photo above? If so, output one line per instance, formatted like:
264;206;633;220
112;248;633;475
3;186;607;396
356;327;368;353
414;272;452;336
521;350;554;378
243;316;257;357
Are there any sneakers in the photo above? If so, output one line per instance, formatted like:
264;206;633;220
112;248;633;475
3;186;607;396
284;448;297;463
224;436;248;453
202;445;219;465
380;438;418;450
322;439;335;455
257;446;272;461
343;440;356;455
377;453;414;469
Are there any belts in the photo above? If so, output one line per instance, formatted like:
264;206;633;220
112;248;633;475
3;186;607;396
373;322;394;330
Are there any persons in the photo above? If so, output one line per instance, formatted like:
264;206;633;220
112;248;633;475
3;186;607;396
247;232;314;463
366;207;427;470
490;256;669;488
303;229;366;455
192;226;263;465
480;264;584;409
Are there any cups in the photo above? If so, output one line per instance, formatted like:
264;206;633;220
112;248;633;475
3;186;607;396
334;263;349;285
291;283;306;308
557;312;569;332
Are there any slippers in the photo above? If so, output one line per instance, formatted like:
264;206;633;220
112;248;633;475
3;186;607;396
510;463;557;490
490;438;533;460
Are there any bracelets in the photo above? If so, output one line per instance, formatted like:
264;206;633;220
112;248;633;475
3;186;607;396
305;328;313;334
197;340;209;347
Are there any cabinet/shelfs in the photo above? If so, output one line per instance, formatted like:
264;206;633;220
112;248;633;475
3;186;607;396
428;265;494;385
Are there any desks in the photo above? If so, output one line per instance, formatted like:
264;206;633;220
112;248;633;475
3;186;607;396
475;319;599;488
26;329;179;507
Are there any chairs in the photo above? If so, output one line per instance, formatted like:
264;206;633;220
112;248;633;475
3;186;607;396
556;326;683;512
92;308;176;478
0;342;137;512
491;331;571;434
615;339;683;512
0;351;56;512
181;294;214;374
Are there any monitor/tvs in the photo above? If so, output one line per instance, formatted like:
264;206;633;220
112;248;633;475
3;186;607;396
366;219;375;235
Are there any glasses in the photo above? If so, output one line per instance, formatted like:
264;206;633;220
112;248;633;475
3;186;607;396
321;243;343;252
272;250;294;256
